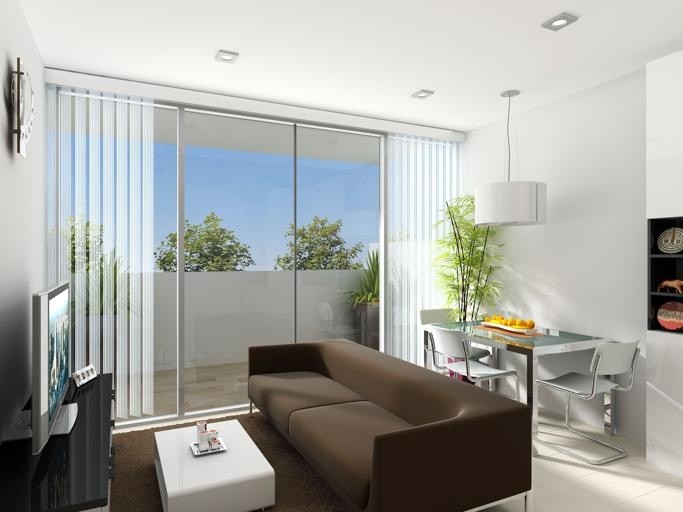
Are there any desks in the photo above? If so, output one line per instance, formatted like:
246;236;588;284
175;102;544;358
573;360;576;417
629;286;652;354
2;372;116;511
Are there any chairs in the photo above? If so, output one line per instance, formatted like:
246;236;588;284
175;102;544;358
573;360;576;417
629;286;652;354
419;308;489;379
534;339;641;465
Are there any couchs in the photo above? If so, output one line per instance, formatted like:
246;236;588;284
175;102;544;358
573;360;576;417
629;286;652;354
248;338;531;512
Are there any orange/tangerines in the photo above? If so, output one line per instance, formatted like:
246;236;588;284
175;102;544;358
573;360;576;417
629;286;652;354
485;314;534;329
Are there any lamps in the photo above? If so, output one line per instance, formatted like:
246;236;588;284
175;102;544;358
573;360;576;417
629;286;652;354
474;90;547;228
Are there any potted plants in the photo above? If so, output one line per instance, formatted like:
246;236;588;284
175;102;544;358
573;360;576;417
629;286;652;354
432;196;506;387
337;248;379;351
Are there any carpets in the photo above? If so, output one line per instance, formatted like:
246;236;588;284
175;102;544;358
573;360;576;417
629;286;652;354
109;411;351;512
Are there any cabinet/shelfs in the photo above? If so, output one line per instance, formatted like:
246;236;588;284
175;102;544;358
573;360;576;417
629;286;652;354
646;217;683;334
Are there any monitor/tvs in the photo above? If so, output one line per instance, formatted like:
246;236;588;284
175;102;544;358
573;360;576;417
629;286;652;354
2;281;79;455
